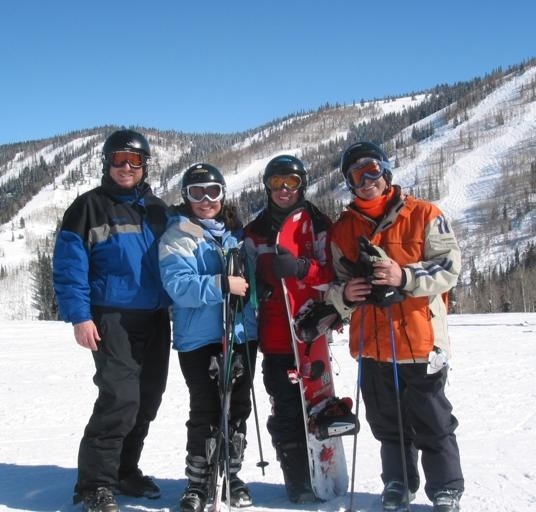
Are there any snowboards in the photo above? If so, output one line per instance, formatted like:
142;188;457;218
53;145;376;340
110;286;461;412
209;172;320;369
276;207;359;500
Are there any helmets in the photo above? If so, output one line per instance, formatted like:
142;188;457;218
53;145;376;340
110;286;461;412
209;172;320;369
103;130;150;181
341;142;392;194
181;163;225;205
263;155;307;194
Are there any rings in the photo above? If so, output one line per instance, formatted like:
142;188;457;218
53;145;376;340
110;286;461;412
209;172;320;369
382;271;387;279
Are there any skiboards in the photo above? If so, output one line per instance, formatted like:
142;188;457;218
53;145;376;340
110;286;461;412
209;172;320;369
203;248;244;512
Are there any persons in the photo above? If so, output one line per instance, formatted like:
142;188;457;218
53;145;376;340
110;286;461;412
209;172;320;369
156;163;260;511
53;130;169;511
324;142;464;511
241;155;334;503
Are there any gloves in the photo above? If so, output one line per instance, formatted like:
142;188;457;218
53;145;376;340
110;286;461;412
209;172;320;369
271;245;308;280
358;234;406;307
339;256;378;305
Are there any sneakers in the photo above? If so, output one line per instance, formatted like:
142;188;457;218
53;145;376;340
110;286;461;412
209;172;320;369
83;486;120;512
179;484;209;512
285;478;314;504
431;487;462;512
119;468;161;498
223;474;253;506
381;478;416;511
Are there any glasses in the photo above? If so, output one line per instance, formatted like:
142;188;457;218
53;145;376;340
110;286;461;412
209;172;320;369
181;182;226;203
263;170;306;191
345;158;393;191
102;151;151;169
